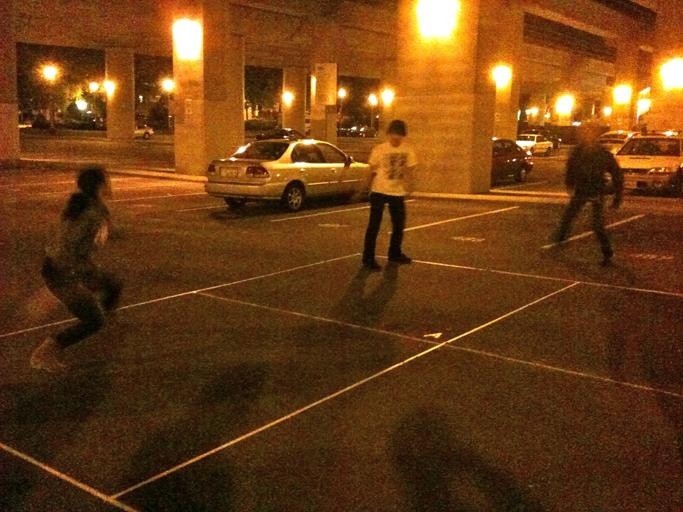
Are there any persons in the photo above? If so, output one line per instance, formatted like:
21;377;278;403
357;117;414;275
538;122;627;277
28;161;131;376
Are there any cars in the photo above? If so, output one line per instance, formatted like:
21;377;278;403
202;126;375;214
134;125;154;139
597;130;682;196
491;129;563;185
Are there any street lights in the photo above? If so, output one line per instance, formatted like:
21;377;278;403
368;94;377;124
42;64;57;128
88;82;99;120
163;80;173;127
338;87;346;121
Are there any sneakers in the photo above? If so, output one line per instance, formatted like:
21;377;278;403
360;254;412;272
30;338;71;374
